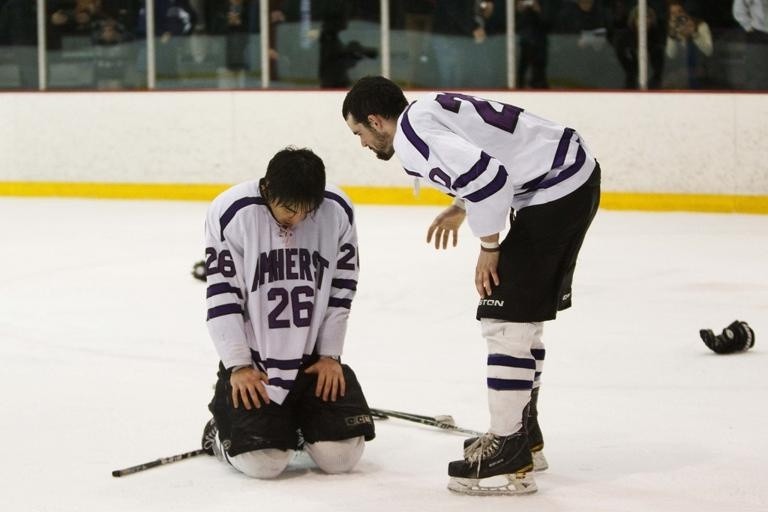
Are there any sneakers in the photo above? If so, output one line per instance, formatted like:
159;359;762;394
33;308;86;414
200;416;220;455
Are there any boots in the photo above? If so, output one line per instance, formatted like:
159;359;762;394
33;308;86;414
448;401;534;477
463;386;544;454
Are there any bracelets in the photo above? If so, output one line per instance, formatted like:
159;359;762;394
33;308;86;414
451;199;466;212
231;365;246;373
478;241;500;253
320;355;340;362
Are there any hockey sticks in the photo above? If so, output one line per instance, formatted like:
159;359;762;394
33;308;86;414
112;409;388;477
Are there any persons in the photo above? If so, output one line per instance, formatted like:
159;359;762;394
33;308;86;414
342;76;602;480
0;2;765;92
202;146;376;478
